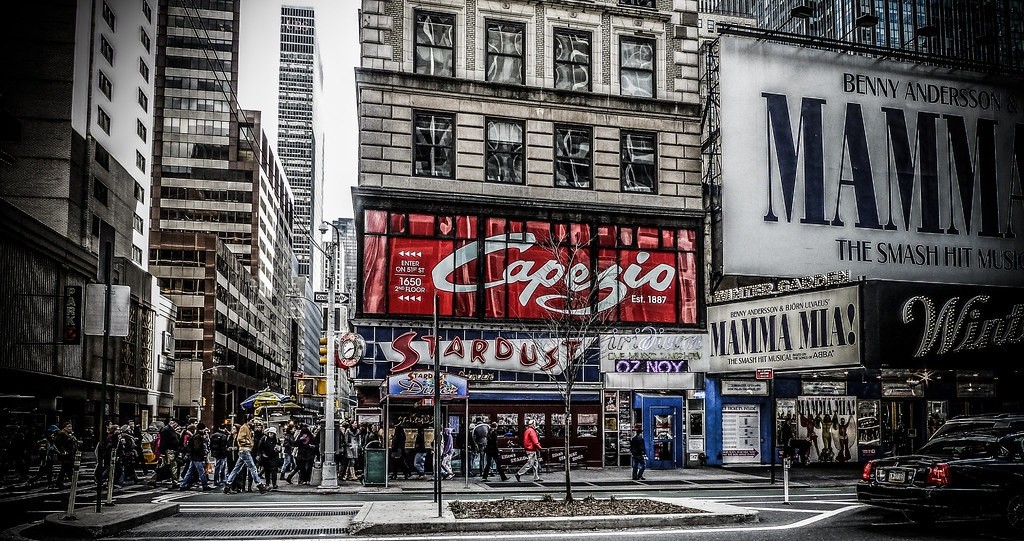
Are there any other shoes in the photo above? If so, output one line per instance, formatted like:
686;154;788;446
203;486;213;491
48;484;67;490
638;476;645;480
258;474;264;479
273;485;278;489
515;473;520;482
503;477;511;481
534;479;543;482
482;480;491;482
302;481;310;485
446;474;455;480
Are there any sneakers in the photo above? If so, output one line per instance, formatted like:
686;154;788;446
223;485;237;494
256;483;273;494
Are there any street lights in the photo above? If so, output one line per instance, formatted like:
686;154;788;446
317;220;341;490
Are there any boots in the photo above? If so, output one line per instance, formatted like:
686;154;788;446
343;467;358;481
298;478;303;486
280;472;287;480
285;474;293;484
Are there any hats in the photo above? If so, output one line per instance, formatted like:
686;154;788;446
187;425;196;430
172;418;181;426
220;425;226;429
120;425;130;433
197;422;205;430
267;427;277;436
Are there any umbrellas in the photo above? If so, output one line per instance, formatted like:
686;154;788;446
240;389;302;428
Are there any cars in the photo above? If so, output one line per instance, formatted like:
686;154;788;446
856;425;1024;537
926;411;1024;443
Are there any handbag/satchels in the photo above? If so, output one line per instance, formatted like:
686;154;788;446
155;464;173;481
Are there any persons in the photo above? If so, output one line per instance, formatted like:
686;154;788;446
798;410;854;462
781;417;797;458
629;428;647;480
429;426;455;482
0;415;426;493
482;422;511;482
514;419;543;482
